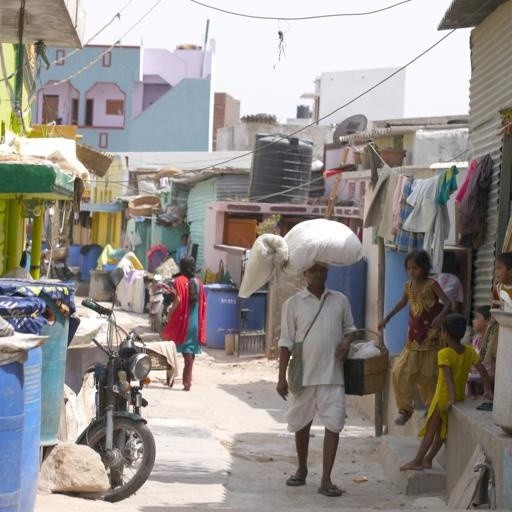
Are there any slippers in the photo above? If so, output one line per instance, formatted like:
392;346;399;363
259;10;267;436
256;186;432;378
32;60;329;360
286;475;306;486
318;485;342;497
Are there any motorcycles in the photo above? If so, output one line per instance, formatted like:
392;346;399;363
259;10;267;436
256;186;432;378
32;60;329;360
27;238;71;283
74;297;158;502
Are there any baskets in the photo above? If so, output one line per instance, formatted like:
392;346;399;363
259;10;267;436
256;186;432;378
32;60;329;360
342;327;390;397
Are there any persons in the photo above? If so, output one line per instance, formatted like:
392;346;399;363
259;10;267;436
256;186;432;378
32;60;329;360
471;305;491;355
400;314;493;471
481;252;512;381
378;249;452;425
162;256;207;391
276;263;358;497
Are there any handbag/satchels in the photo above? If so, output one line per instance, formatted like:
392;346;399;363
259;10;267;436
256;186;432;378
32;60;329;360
287;342;303;394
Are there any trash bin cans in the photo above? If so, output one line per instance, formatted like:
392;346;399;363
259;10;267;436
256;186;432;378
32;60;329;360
203;285;268;350
77;243;102;282
65;244;80;267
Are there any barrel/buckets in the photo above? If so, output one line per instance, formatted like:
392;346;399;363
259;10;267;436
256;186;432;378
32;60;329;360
89;268;110;301
0;278;103;512
70;244;102;280
202;284;267;348
